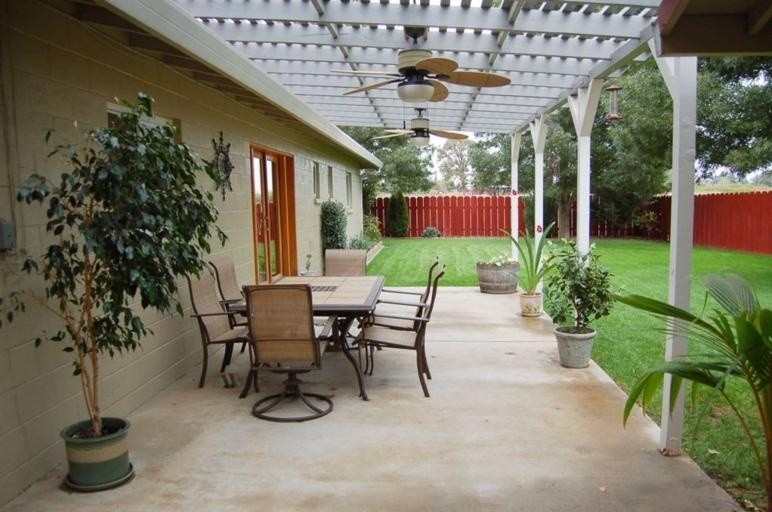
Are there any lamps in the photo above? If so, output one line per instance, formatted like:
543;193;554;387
397;75;434;103
409;130;430;146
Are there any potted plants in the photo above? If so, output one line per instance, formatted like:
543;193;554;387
0;91;231;487
476;221;627;369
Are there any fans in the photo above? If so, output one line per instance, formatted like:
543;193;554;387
372;108;468;139
330;26;511;102
